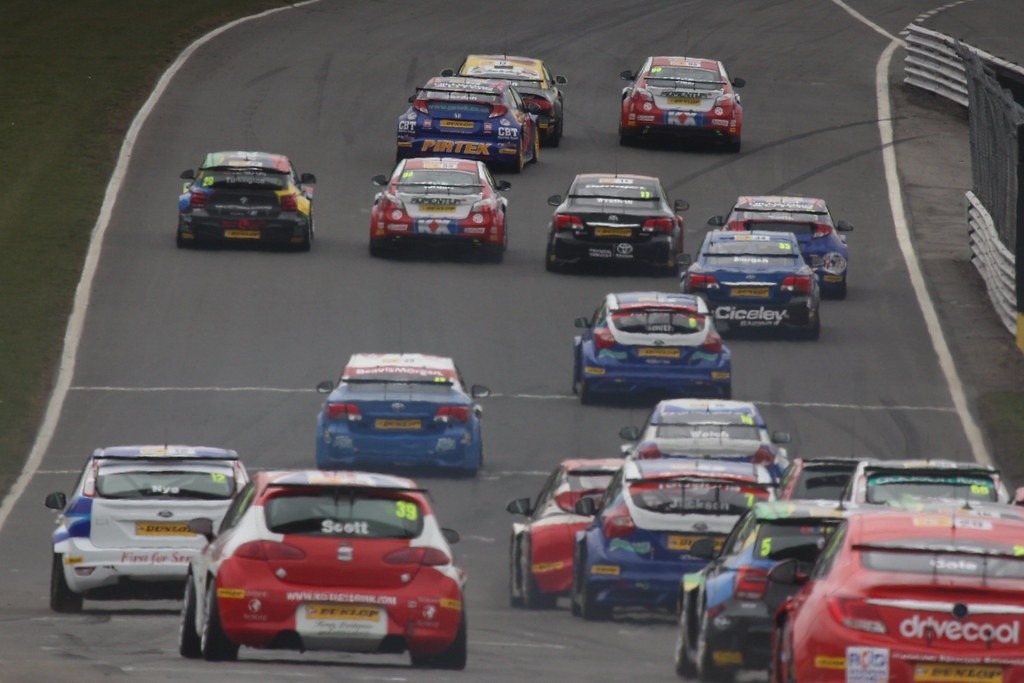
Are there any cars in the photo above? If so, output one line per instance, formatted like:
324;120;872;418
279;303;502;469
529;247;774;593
847;458;1010;514
569;290;735;398
570;454;778;618
505;456;627;608
773;500;1024;683
396;76;542;172
669;497;883;683
615;55;747;152
772;458;861;503
365;156;514;264
708;195;856;296
177;151;318;251
174;469;470;672
314;350;492;477
620;396;794;470
542;174;692;274
39;444;251;615
441;55;567;145
680;226;822;340
